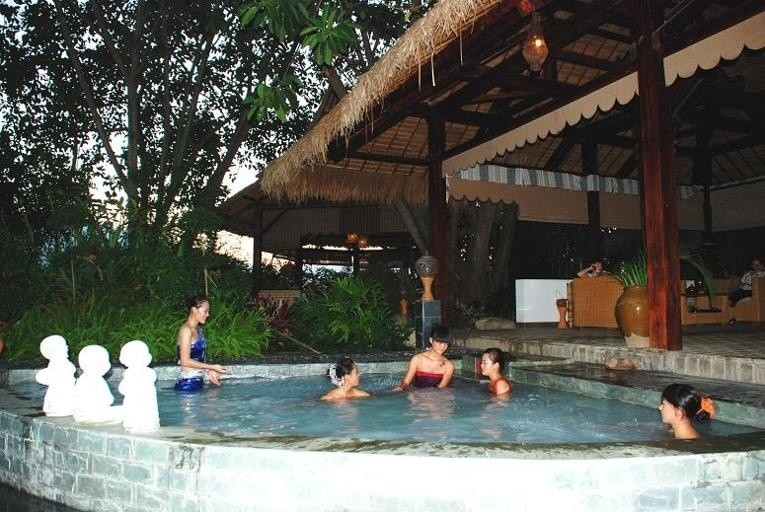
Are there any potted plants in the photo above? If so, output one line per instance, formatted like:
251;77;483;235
608;237;718;349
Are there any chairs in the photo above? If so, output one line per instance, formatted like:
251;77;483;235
568;275;625;330
697;273;765;326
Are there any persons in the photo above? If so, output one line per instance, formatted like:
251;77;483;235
728;257;765;306
176;294;232;394
320;358;370;402
577;260;610;278
390;324;455;392
478;346;513;394
590;382;717;442
31;334;77;417
74;342;124;423
113;338;160;432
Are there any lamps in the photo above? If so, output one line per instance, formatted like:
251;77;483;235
520;1;550;72
